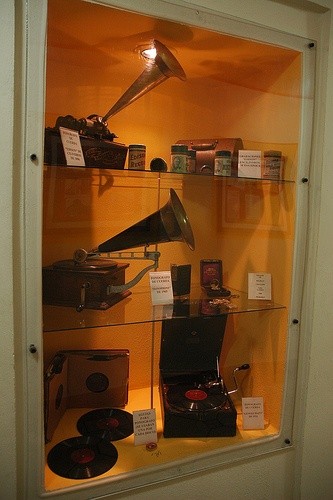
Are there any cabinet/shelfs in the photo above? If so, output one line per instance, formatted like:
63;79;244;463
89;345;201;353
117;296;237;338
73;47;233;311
39;159;294;334
